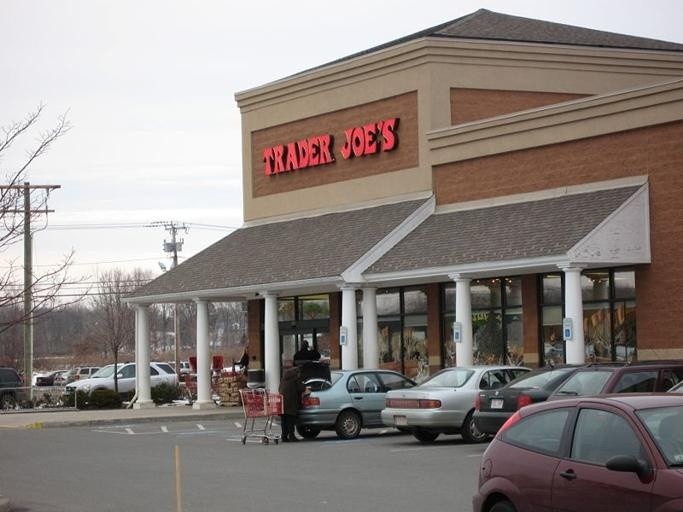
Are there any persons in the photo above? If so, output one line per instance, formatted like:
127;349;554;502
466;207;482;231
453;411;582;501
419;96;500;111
240;347;250;376
278;341;332;442
381;353;398;370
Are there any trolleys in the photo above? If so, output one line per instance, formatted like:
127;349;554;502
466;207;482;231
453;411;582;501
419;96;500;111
238;388;283;445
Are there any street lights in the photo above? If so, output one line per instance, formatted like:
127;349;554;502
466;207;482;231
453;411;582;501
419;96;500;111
157;262;180;387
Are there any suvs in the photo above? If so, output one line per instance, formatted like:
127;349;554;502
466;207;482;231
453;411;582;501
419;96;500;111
0;366;27;410
547;359;682;401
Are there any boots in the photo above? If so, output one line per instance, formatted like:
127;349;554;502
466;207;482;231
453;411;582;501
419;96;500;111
281;430;301;442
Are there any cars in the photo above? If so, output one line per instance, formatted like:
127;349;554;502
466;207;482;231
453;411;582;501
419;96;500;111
665;381;683;394
32;361;196;400
475;389;682;511
379;365;535;445
298;369;417;441
473;364;583;435
